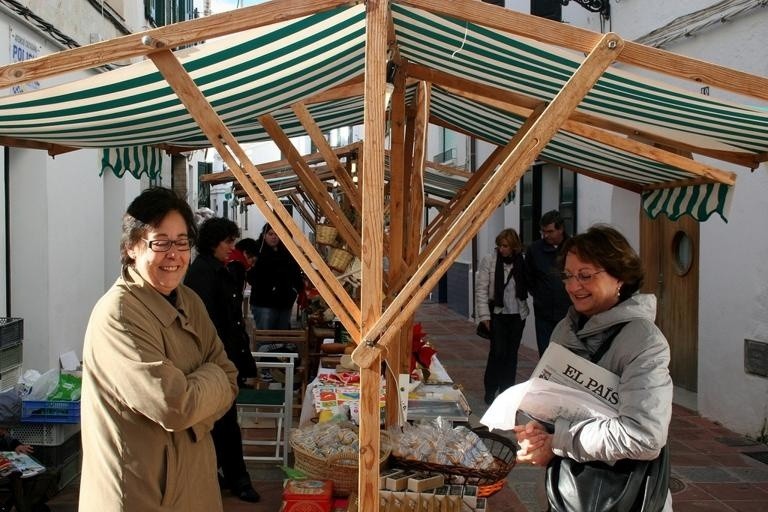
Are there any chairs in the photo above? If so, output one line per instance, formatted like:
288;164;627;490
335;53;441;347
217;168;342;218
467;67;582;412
250;326;309;420
233;352;299;472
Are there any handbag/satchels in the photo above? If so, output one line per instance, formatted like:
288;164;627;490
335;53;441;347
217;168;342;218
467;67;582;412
476;320;491;339
543;418;670;511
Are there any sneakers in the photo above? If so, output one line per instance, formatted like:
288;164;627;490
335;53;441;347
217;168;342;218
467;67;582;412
217;470;260;503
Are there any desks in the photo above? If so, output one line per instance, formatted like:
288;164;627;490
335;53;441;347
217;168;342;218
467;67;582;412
285;334;527;512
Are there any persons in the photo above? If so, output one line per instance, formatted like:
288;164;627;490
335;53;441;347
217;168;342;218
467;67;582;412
473;228;529;404
0;428;51;511
521;209;580;358
224;213;300;379
184;216;260;503
515;221;677;511
76;184;239;512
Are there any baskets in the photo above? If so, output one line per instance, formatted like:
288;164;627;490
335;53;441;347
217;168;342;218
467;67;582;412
395;422;518;498
288;421;390;500
315;222;337;244
325;247;355;272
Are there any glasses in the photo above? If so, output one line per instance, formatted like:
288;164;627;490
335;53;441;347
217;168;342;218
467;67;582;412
138;234;194;252
559;270;606;285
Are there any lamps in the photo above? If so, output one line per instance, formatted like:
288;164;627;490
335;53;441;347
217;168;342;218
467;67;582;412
558;0;611;34
